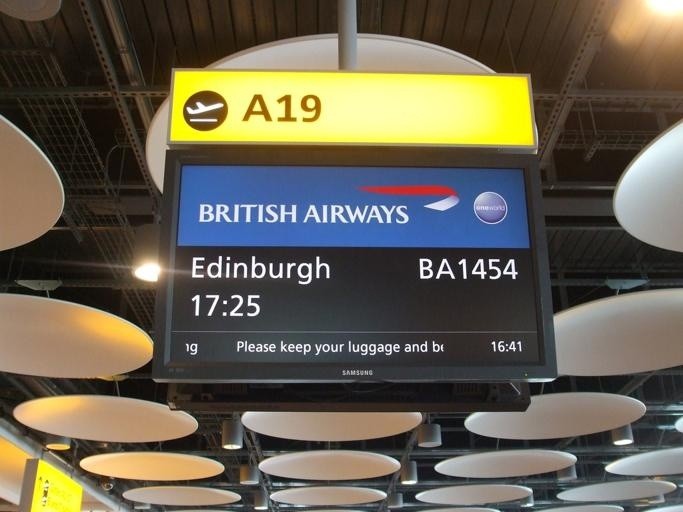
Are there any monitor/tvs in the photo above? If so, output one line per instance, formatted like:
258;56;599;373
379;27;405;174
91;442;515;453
152;145;559;414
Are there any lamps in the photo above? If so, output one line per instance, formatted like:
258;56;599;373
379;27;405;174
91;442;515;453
402;456;417;486
134;500;150;510
415;412;442;448
520;490;534;507
557;461;576;481
222;413;243;451
240;455;259;485
388;481;403;508
612;422;634;446
254;489;268;510
46;433;71;450
131;223;161;283
647;494;665;504
